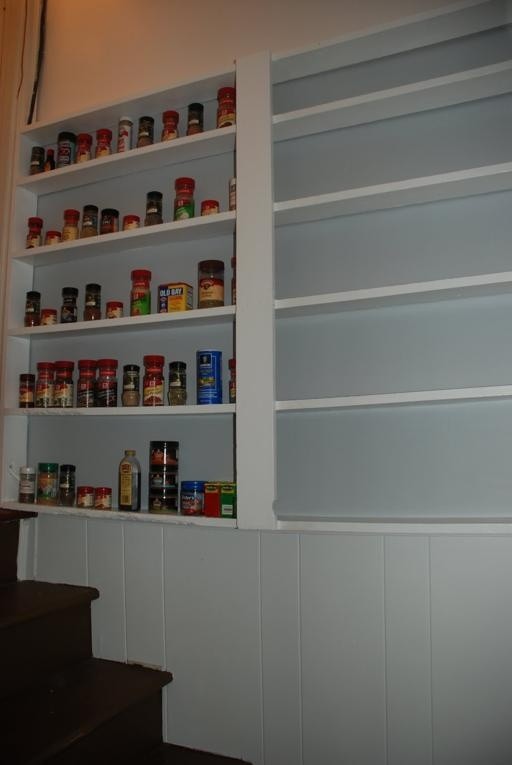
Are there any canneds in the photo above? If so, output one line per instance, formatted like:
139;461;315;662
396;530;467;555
149;440;207;515
123;215;140;230
77;486;94;507
40;309;57;326
201;200;219;216
106;302;123;319
45;231;61;245
95;487;112;509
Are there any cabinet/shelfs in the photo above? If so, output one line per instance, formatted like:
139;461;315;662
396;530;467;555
2;52;259;528
259;1;510;534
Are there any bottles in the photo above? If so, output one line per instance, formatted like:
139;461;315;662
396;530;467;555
174;176;196;222
76;86;236;164
229;178;236;210
19;466;36;503
60;464;76;506
63;205;99;241
26;216;44;248
119;449;141;511
25;290;42;327
129;269;152;316
227;359;236;403
44;149;56;171
196;257;236;309
145;191;163;225
84;283;102;321
20;354;187;408
61;287;79;323
